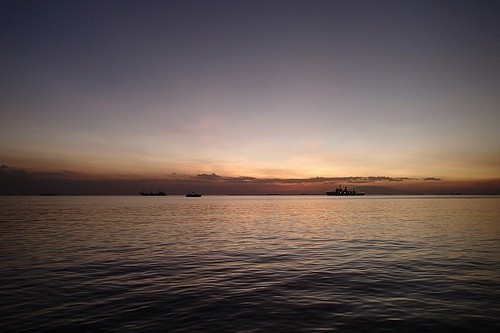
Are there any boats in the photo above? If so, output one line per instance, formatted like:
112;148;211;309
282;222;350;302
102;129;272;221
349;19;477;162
186;191;202;197
140;187;167;196
326;185;365;196
